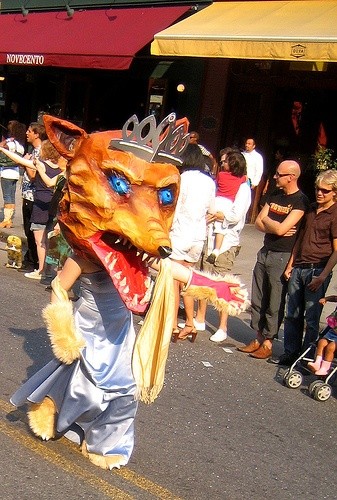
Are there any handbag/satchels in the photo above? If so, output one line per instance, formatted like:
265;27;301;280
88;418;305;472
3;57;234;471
0;140;23;167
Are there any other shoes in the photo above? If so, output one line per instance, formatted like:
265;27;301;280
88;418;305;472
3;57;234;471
24;269;46;279
210;329;227;342
46;272;52;278
178;318;205;331
34;264;39;269
238;340;260;353
249;346;273;358
18;265;34;272
267;354;290;365
295;360;310;376
206;250;220;264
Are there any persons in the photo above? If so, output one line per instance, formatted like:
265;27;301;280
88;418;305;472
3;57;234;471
267;169;337;366
178;147;251;343
188;131;216;163
201;151;247;264
237;160;310;359
241;139;264;224
167;143;216;344
307;296;337;375
0;120;67;280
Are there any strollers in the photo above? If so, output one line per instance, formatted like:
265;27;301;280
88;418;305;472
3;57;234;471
279;295;337;401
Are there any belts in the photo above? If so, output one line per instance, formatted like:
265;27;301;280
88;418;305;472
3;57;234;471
299;264;325;269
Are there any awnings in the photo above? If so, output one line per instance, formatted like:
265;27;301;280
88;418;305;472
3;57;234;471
151;1;337;62
0;5;191;70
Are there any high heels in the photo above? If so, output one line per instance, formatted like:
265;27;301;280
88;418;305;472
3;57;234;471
171;328;180;343
0;220;12;229
178;325;197;343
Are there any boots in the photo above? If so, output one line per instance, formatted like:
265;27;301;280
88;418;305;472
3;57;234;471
308;356;322;372
315;360;332;375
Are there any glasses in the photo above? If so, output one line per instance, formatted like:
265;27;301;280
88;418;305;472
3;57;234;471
276;172;295;178
316;187;334;193
190;137;197;139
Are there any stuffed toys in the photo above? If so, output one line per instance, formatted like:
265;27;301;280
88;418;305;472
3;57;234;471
10;113;252;469
